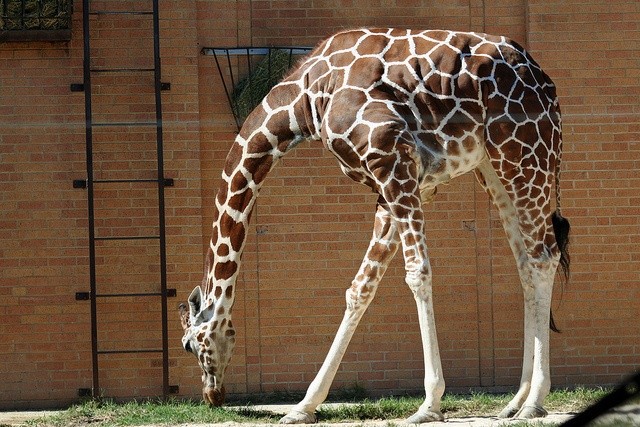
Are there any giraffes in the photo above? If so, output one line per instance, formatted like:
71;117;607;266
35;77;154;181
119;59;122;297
178;26;571;425
